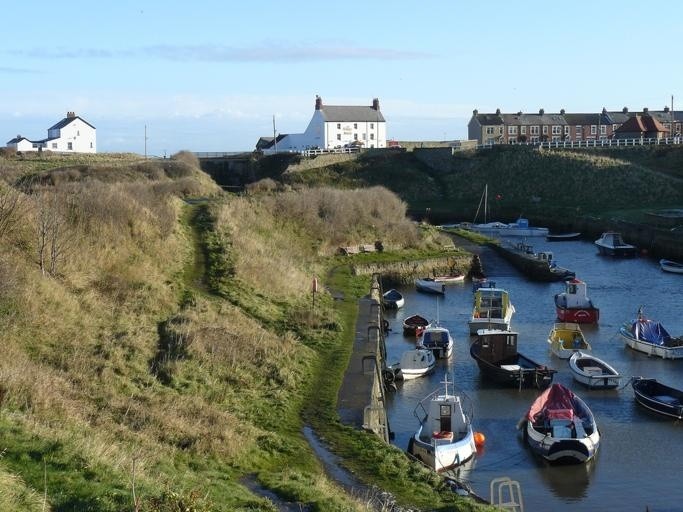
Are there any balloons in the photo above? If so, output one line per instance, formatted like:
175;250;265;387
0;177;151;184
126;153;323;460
473;432;485;445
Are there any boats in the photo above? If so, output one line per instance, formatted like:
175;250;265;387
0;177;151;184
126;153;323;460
416;272;445;294
545;320;591;360
439;184;682;281
382;289;404;310
416;295;452;361
630;375;682;421
468;279;515;334
570;351;619;390
618;304;681;361
388;349;435;381
552;277;599;324
513;381;600;465
407;371;485;486
470;326;556;390
402;314;431;337
